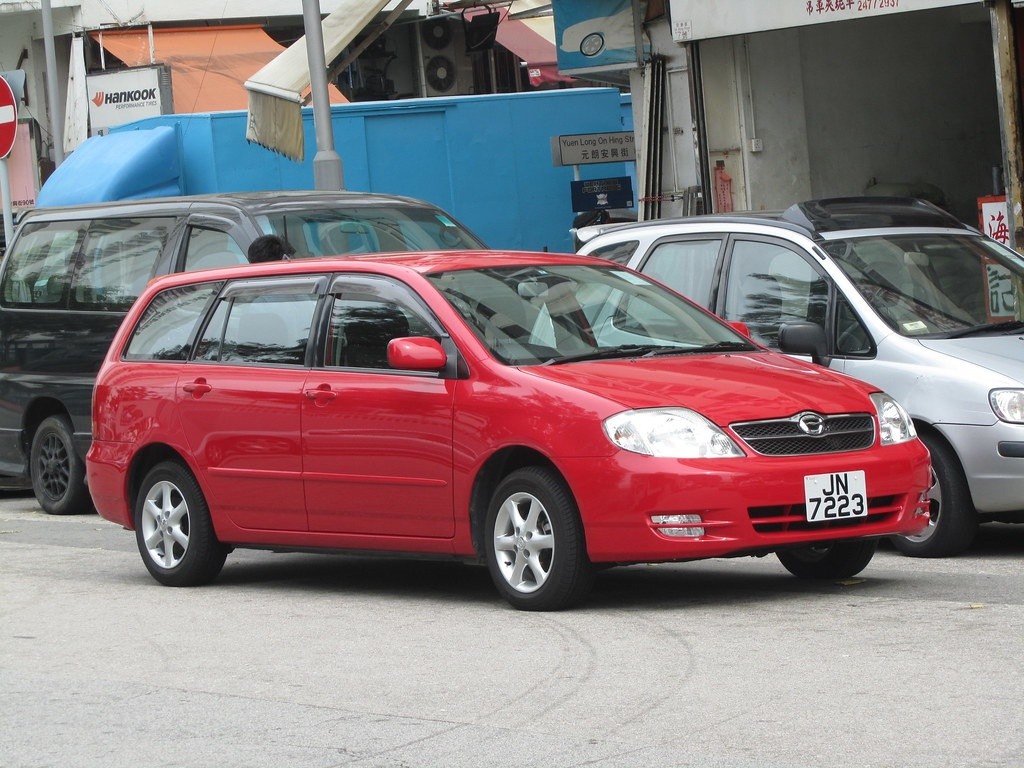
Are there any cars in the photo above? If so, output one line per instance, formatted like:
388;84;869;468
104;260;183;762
85;248;934;613
528;195;1024;560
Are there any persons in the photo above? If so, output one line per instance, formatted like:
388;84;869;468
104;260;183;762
249;235;296;263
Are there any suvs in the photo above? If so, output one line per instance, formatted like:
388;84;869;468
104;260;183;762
1;189;491;516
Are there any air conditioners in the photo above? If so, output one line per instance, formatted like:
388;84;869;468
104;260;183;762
410;18;459;97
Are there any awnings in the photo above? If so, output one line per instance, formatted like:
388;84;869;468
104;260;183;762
244;1;415;162
91;23;353;115
443;0;633;86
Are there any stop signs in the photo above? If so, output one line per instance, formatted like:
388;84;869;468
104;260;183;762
0;77;18;161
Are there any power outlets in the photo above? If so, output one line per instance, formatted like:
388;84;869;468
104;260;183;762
752;139;763;153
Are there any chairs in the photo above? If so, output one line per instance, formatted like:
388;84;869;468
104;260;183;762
732;273;781;348
343;308;410;370
